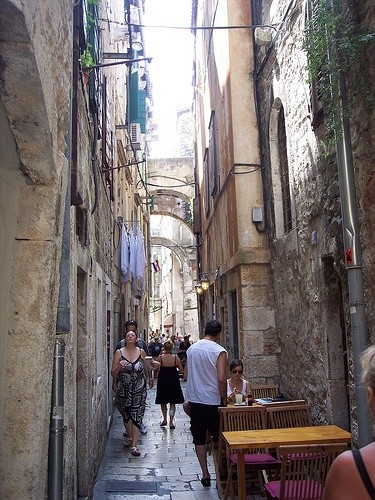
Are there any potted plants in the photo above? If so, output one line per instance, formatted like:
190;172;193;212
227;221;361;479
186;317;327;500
80;50;95;86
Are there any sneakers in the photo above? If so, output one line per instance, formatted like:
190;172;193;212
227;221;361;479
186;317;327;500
123;429;128;436
130;446;141;456
124;438;132;447
141;424;147;434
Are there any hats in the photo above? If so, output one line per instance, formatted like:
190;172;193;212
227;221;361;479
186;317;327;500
125;320;137;327
183;334;191;339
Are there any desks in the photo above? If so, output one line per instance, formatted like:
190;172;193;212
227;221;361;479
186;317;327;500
221;425;351;500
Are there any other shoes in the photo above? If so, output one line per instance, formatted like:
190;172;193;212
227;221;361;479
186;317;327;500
170;425;175;429
160;421;167;426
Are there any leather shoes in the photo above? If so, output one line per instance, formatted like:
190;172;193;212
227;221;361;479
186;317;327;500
201;477;211;486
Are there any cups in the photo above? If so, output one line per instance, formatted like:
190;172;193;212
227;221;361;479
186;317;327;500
235;392;243;404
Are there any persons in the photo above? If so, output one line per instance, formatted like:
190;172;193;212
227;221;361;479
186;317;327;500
327;346;375;500
111;320;195;456
179;321;226;486
226;359;253;403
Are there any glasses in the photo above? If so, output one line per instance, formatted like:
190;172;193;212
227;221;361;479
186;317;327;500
232;370;244;374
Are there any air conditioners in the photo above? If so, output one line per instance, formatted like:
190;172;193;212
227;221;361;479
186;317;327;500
122;123;142;151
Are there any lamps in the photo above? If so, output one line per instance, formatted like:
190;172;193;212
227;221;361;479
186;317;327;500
193;279;204;295
200;272;210;291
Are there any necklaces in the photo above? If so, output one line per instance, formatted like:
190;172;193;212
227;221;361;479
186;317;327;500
229;378;241;388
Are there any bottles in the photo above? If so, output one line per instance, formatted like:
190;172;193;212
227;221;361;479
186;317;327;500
247;389;252;406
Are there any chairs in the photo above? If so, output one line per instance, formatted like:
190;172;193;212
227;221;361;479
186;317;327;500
268;403;329;480
218;406;282;499
264;443;347;500
251;384;279;400
